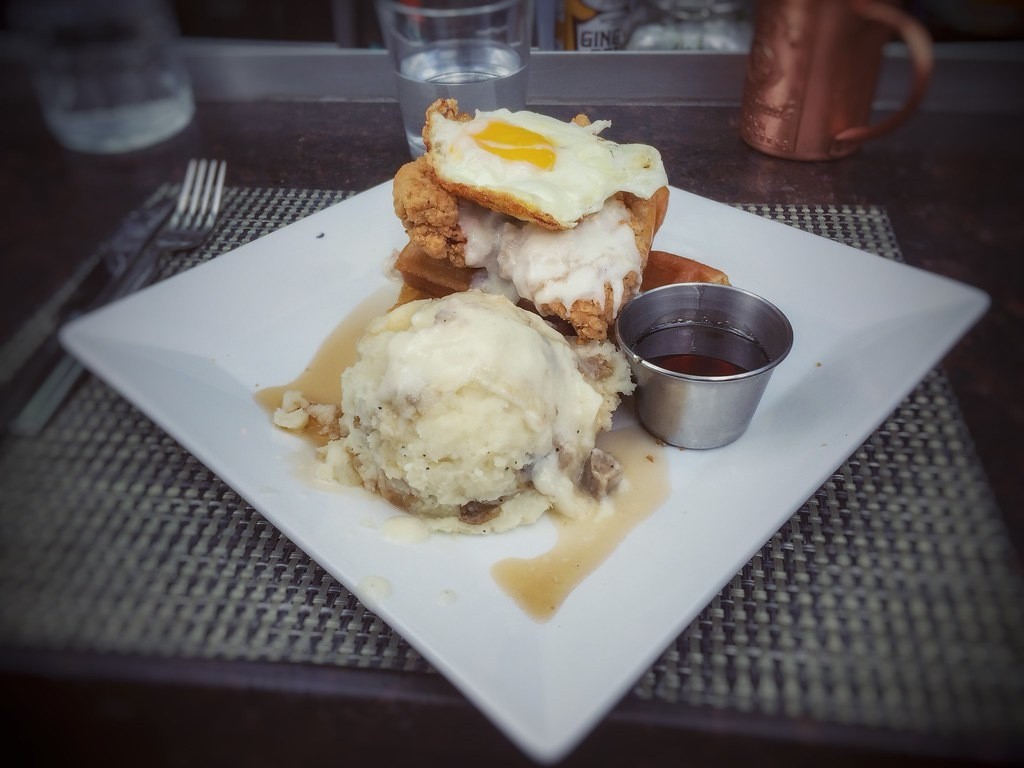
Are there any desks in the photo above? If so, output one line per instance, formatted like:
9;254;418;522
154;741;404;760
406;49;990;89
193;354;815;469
0;94;1024;768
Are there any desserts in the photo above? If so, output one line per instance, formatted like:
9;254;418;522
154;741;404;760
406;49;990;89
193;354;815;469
339;293;598;531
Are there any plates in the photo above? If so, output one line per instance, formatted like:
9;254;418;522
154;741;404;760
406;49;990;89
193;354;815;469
55;175;990;762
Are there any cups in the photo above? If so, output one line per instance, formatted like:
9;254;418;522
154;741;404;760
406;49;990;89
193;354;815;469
735;0;937;163
382;0;533;161
614;282;794;451
0;0;197;157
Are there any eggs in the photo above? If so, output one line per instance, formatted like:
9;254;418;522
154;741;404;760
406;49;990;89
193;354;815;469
427;106;666;229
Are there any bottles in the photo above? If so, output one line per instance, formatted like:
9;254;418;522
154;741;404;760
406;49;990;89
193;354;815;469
626;0;752;53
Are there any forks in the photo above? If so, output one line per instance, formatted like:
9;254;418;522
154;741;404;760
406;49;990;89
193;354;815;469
11;159;227;440
